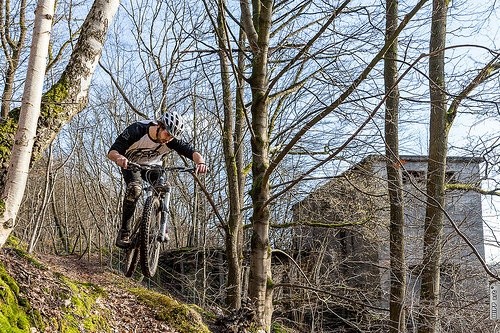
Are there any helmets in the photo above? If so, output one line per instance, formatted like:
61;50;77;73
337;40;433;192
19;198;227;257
157;110;185;141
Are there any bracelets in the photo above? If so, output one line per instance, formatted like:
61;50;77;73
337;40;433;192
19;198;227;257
198;163;205;165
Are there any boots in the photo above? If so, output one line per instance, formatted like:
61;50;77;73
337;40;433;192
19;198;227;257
114;199;136;248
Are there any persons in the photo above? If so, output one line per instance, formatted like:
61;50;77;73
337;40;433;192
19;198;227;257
107;111;209;249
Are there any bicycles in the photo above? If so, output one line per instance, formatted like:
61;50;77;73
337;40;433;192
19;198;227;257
120;161;211;277
